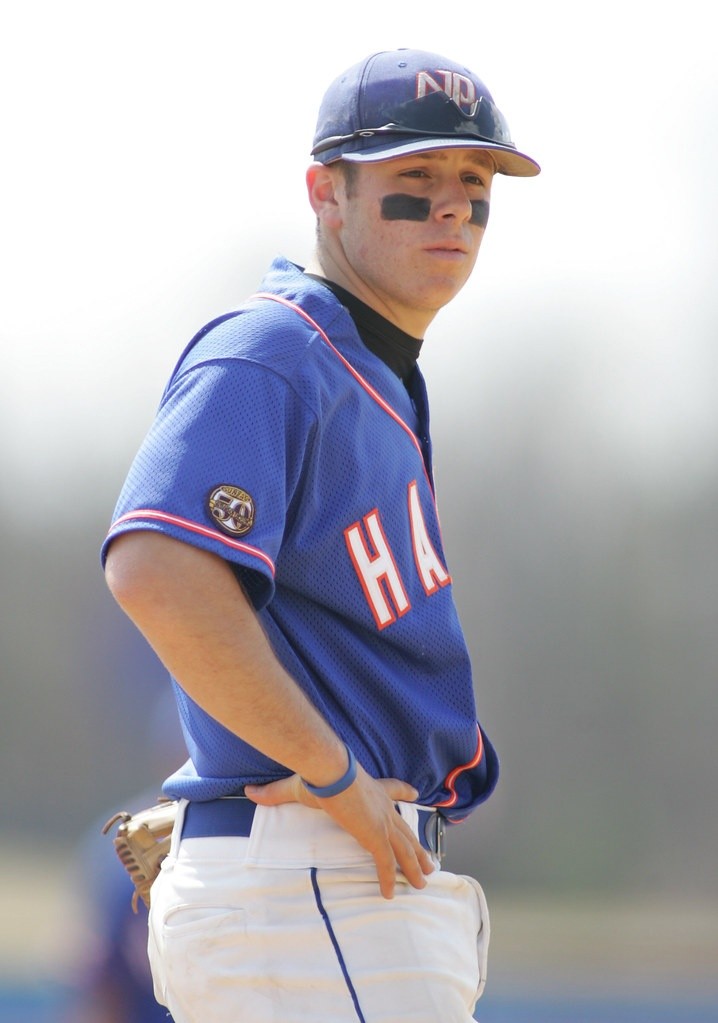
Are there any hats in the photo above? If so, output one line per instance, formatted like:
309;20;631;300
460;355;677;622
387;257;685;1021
311;48;542;178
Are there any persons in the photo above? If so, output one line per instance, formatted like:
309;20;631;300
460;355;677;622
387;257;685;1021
104;51;540;1023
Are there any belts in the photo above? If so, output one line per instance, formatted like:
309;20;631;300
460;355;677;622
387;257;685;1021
180;792;444;863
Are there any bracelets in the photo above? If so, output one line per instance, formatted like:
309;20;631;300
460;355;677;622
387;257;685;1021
301;742;356;798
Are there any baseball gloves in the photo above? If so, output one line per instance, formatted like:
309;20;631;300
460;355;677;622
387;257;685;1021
108;797;184;893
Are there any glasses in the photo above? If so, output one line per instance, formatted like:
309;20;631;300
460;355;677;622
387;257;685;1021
311;90;515;147
